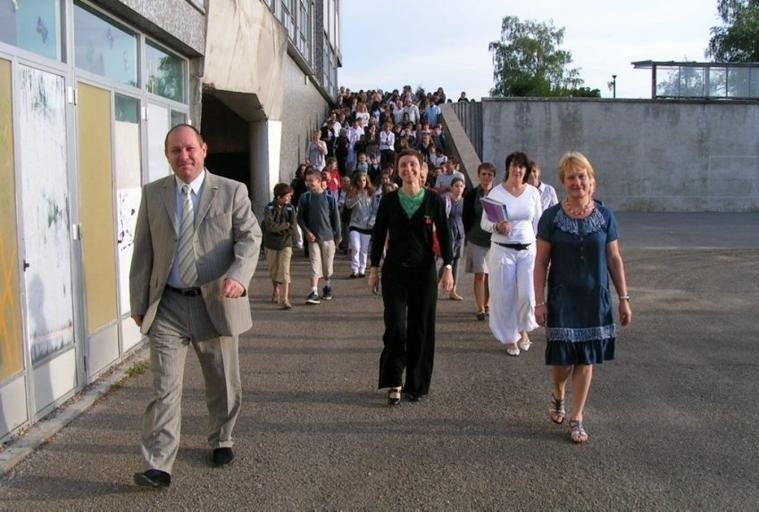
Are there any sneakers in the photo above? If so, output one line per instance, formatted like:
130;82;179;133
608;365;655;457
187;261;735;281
477;304;490;322
350;273;359;278
358;273;365;277
279;297;292;309
450;294;463;301
305;292;320;304
323;285;333;300
272;289;280;304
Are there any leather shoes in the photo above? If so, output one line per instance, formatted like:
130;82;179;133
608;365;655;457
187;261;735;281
507;346;520;356
406;394;417;402
389;384;403;405
133;469;171;488
213;446;234;466
519;339;533;351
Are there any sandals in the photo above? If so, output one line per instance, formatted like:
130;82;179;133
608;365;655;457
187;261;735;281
569;418;589;444
549;392;567;424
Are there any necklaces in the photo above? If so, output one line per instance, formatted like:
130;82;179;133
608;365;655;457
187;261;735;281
565;199;592;216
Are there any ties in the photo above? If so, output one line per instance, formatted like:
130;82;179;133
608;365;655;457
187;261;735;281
177;185;198;287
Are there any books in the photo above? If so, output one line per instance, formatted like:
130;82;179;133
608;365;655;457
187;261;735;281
480;197;511;236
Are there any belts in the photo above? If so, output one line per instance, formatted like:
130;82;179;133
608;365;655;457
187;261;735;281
166;283;202;297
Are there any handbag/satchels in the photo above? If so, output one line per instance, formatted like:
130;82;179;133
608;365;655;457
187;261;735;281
432;193;442;256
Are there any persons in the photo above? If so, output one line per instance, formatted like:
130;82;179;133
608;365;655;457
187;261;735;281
129;124;263;487
262;85;467;308
480;151;543;356
525;160;559;212
534;150;633;442
462;163;496;321
369;149;455;405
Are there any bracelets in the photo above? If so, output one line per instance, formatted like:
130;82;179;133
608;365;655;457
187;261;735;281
619;295;631;300
534;301;546;308
